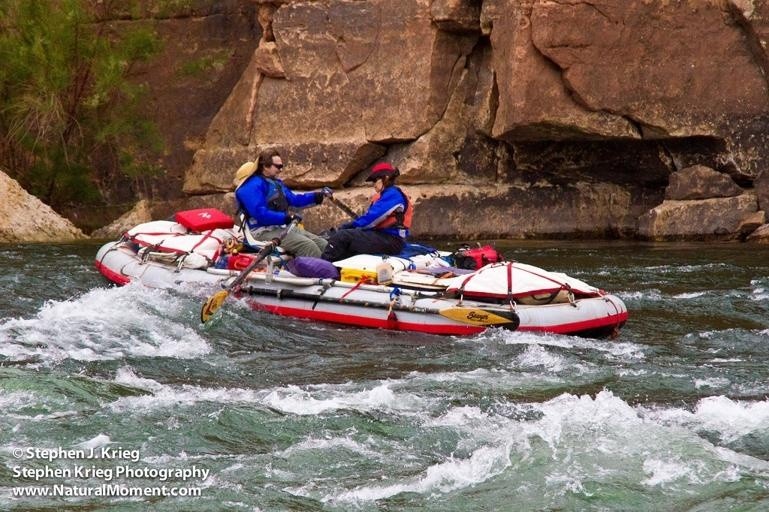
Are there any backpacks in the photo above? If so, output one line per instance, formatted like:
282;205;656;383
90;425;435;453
455;245;505;271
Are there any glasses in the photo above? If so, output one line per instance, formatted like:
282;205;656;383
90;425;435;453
373;178;380;183
272;163;283;169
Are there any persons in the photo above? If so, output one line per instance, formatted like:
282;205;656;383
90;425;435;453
316;161;417;265
234;144;330;263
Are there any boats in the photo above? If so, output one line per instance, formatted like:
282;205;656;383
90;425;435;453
94;219;627;338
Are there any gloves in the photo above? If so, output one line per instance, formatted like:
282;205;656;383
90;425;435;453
321;186;333;197
286;213;303;226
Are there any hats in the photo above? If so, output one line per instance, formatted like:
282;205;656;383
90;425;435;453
234;156;260;192
365;163;394;183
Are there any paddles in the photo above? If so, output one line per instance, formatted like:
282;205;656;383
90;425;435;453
200;213;302;324
239;284;520;332
